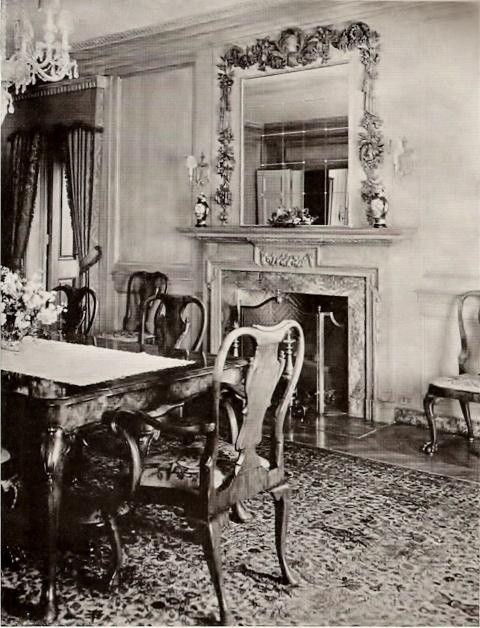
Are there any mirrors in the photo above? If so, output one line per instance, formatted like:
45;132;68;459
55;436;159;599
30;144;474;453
238;58;352;227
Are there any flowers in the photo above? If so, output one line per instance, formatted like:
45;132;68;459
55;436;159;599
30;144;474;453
2;264;61;349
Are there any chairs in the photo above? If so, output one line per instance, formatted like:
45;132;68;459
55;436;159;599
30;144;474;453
121;296;206;463
1;442;37;610
46;283;100;336
420;292;480;455
104;271;167;345
98;320;305;626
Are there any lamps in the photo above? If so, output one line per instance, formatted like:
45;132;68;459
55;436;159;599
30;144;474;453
182;155;198;185
2;0;80;121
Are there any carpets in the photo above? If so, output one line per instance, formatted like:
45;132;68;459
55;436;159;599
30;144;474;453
1;438;478;626
